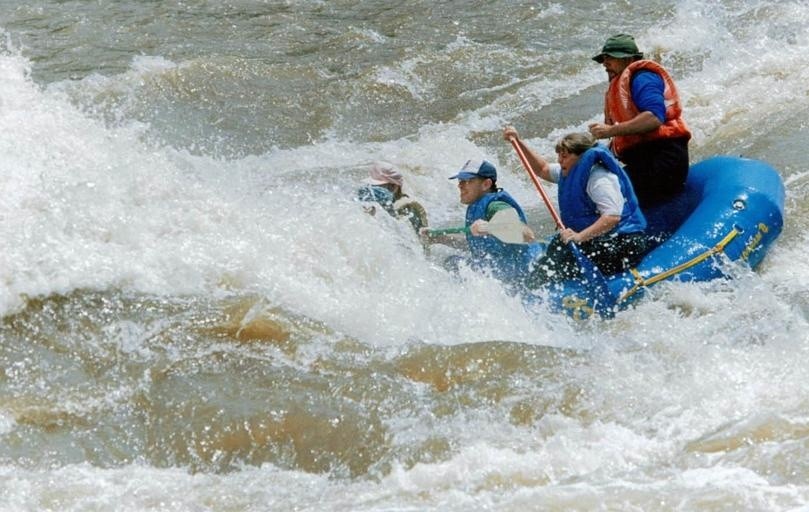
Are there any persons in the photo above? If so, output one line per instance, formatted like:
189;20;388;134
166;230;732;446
359;159;427;229
501;125;647;287
586;33;691;210
418;159;539;287
358;187;418;235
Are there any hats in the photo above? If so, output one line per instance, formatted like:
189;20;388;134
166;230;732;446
362;161;404;187
592;34;644;64
448;158;497;183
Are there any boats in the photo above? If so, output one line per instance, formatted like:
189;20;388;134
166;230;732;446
425;157;785;322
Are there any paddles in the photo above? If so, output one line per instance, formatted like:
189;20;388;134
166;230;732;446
507;132;616;323
421;208;526;246
